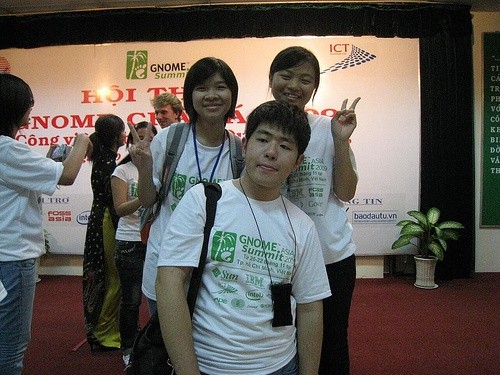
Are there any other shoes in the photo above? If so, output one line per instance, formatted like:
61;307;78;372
123;354;133;366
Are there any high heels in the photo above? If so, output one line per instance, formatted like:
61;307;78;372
87;341;100;352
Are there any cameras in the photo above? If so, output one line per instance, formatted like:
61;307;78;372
51;144;72;162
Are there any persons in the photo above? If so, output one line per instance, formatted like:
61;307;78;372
109;121;159;369
268;46;359;375
128;57;247;375
0;73;93;375
154;99;332;375
83;114;122;351
151;93;183;130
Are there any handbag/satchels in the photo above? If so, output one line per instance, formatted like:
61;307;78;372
138;195;164;244
128;307;194;374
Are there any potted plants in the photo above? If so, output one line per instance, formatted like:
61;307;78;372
391;207;465;286
35;228;51;281
